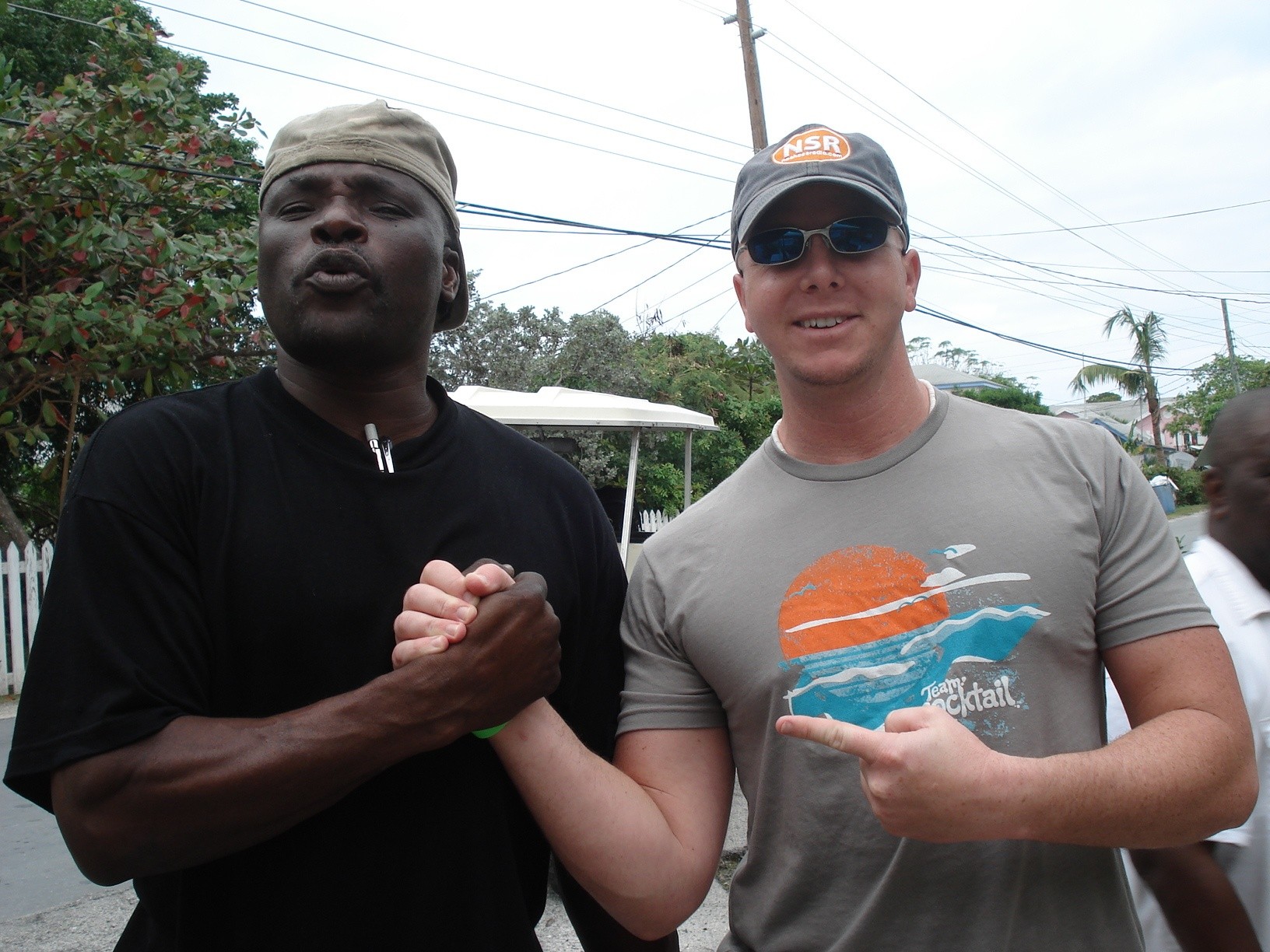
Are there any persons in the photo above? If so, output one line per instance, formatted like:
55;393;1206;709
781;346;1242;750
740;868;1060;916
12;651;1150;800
1113;391;1269;952
389;119;1258;950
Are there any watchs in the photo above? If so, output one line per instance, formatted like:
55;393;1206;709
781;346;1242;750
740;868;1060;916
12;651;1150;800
4;97;676;952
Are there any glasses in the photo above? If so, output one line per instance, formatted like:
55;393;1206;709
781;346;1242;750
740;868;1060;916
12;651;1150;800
736;216;905;274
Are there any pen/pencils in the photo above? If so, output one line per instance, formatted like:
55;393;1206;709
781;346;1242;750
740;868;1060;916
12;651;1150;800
364;422;397;476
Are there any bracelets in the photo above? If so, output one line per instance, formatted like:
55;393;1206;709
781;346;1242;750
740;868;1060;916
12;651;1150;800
471;723;506;738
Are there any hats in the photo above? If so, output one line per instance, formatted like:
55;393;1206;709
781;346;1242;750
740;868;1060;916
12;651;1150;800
731;124;911;270
257;100;469;334
